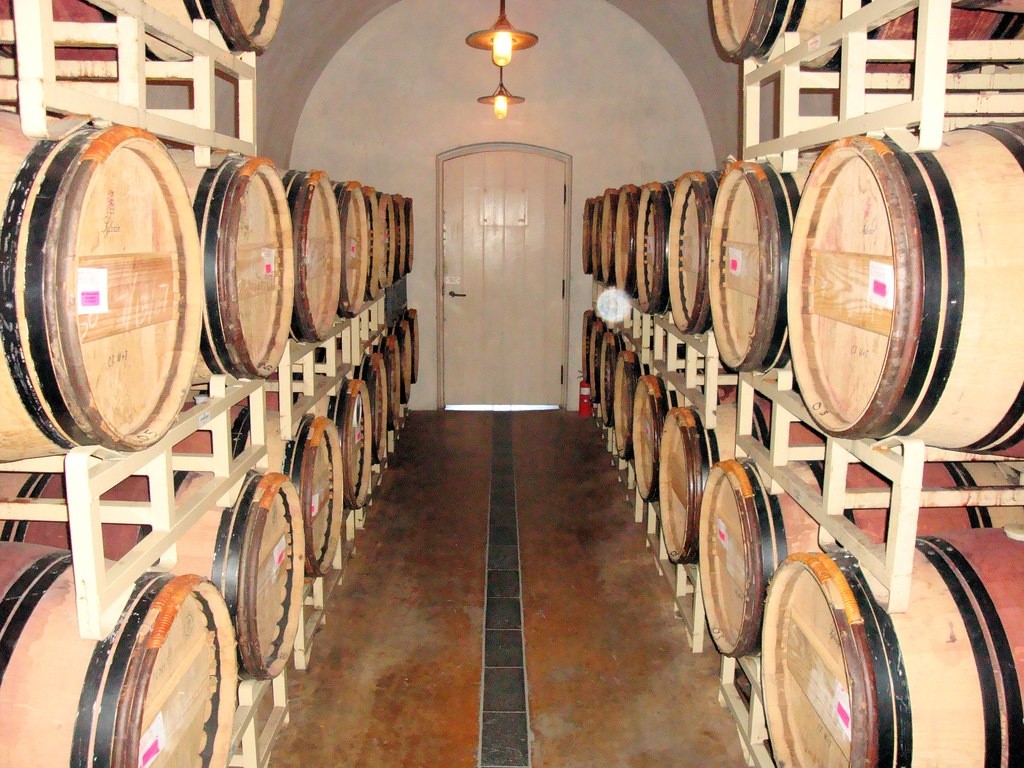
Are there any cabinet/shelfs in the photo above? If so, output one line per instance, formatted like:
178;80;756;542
0;0;402;768
589;0;1024;768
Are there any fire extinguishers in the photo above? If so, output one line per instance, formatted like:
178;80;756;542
577;370;592;417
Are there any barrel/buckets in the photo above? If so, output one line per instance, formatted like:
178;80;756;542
0;0;420;768
582;0;1023;767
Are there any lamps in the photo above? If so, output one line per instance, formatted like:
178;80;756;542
476;67;526;119
465;0;539;66
